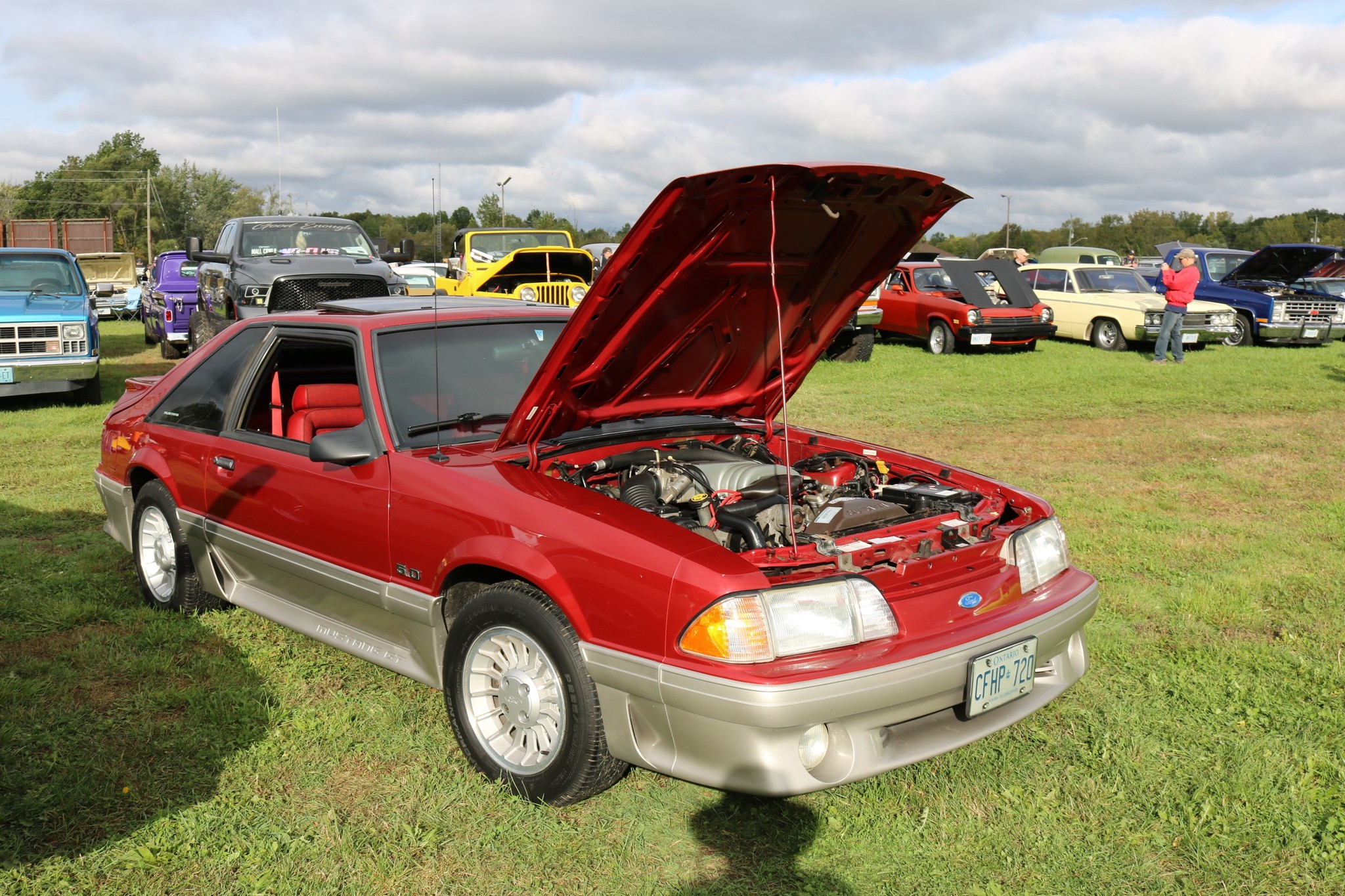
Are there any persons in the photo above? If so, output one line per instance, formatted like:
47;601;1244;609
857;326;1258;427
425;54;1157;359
984;250;996;260
1013;248;1030;268
1149;248;1200;364
1120;249;1139;267
586;247;613;277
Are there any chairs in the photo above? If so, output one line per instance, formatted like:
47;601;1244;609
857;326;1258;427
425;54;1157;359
284;382;366;442
30;278;62;292
111;286;143;322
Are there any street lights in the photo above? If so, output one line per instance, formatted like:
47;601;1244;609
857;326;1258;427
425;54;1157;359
1068;214;1088;247
1001;194;1015;248
497;175;513;252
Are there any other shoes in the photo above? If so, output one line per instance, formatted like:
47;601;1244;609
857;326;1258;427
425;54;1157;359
1171;359;1184;364
1150;359;1166;366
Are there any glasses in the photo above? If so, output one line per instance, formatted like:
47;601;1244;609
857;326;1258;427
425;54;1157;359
602;253;606;256
1179;257;1186;261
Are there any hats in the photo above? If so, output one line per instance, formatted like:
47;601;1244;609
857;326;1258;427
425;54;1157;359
1173;249;1195;258
1128;249;1136;255
601;247;612;255
1016;248;1029;256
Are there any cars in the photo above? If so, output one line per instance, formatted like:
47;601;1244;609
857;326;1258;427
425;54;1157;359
874;258;1058;357
385;226;622;310
827;239;1345;367
90;159;1096;811
987;262;1238;353
0;247;115;408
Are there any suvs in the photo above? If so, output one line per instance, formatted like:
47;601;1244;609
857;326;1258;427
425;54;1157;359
138;249;215;360
186;214;415;357
